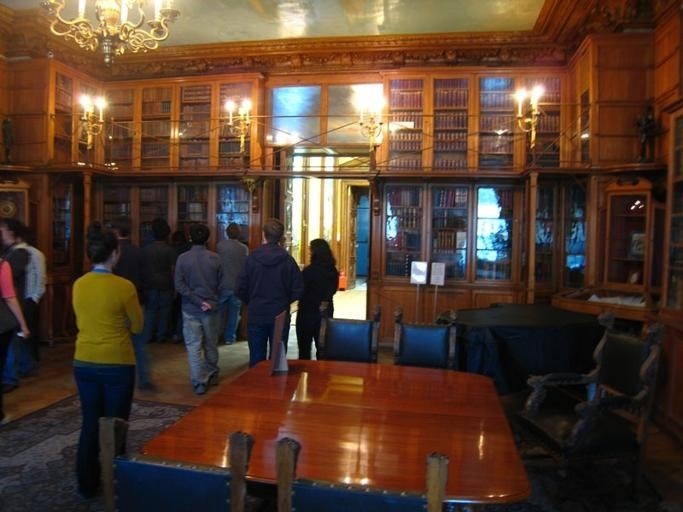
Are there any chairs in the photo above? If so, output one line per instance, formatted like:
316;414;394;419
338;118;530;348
393;305;457;371
518;330;661;512
317;301;381;363
112;417;249;512
276;437;450;512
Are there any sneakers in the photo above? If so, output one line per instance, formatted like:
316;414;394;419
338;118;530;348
195;384;206;394
0;384;18;395
207;376;218;388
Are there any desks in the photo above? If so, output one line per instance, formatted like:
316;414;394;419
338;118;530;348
436;300;646;395
142;357;533;505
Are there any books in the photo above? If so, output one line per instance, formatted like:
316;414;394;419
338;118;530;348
525;80;560;167
388;183;469;280
432;78;469;171
475;187;585;282
388;78;422;171
53;73;251;269
478;79;513;170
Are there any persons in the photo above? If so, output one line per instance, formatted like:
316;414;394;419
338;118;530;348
235;217;305;369
217;223;251;345
71;220;145;500
0;218;50;427
174;224;225;396
89;217;194;390
297;239;340;361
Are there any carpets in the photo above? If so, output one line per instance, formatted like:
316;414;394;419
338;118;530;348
0;391;665;511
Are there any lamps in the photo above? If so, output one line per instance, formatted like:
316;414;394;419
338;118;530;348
78;92;109;150
358;100;383;170
223;99;253;155
38;0;182;69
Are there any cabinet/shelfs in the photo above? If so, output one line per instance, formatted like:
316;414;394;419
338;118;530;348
1;175;73;344
523;180;589;290
602;179;656;296
382;71;562;171
103;71;267;174
92;181;263;343
379;179;517;285
0;58;103;168
562;39;593;168
